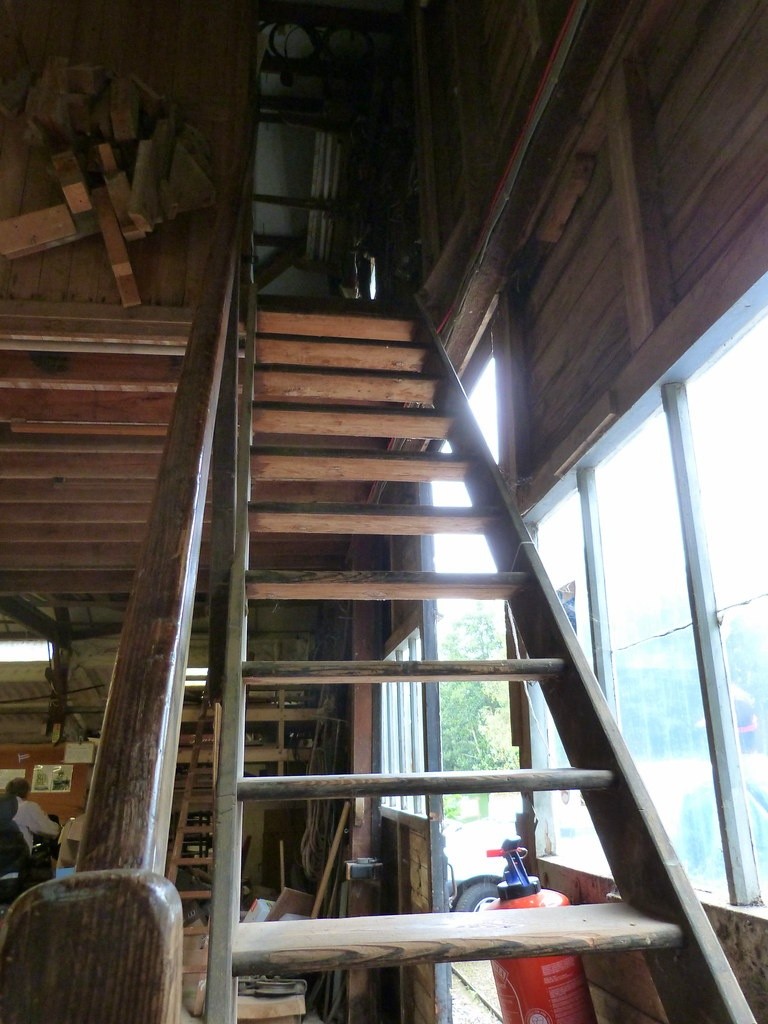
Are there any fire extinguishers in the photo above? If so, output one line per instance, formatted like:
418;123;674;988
483;835;598;1024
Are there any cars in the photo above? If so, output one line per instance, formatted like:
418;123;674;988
442;814;622;912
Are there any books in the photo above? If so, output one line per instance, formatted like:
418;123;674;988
242;899;276;923
239;975;307;996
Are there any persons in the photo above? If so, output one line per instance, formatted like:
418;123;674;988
4;777;62;883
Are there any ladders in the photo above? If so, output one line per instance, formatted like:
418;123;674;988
165;670;217;1019
